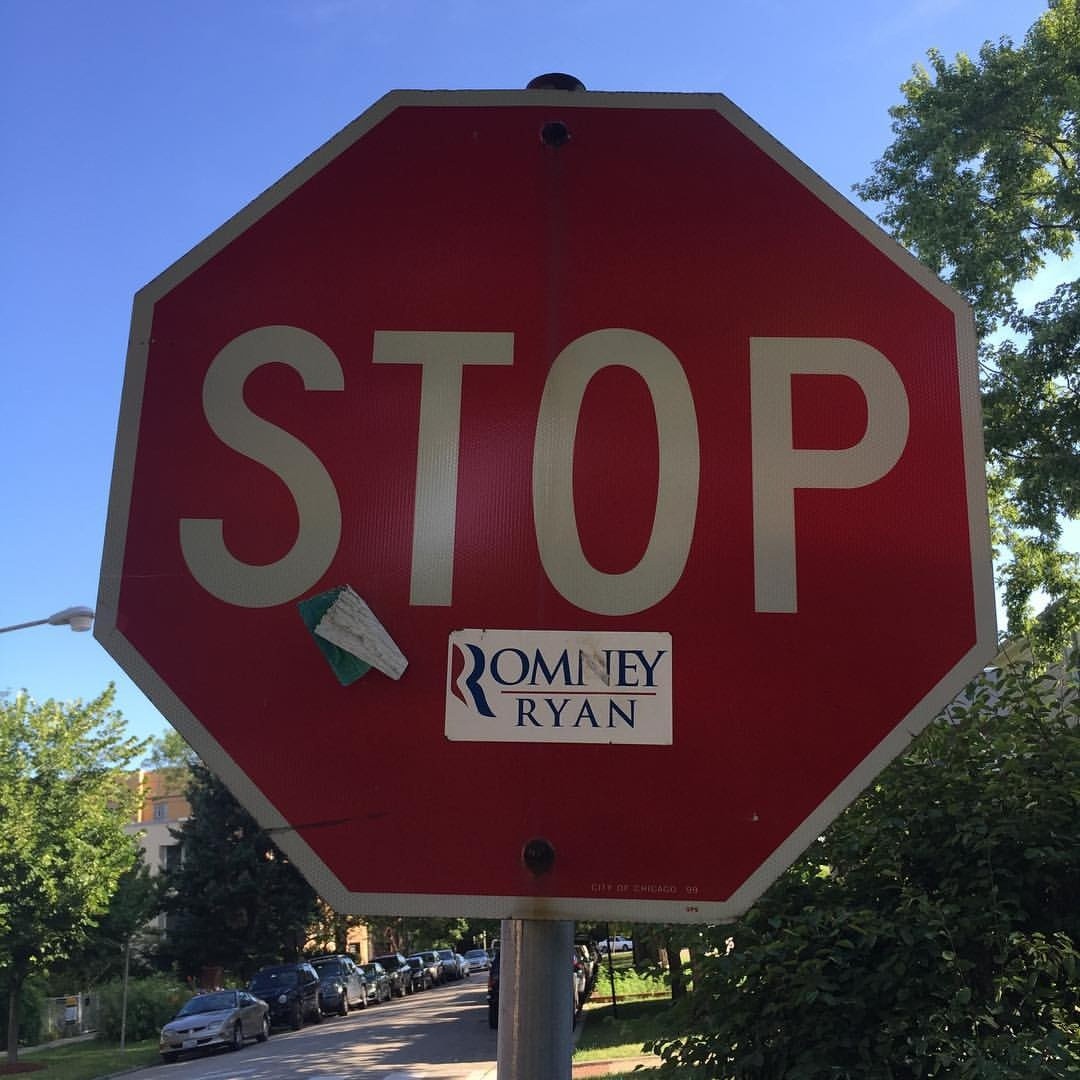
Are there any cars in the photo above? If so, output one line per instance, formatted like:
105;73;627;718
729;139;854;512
356;962;393;1004
455;953;471;978
158;990;271;1064
464;949;488;971
598;937;633;953
405;957;433;992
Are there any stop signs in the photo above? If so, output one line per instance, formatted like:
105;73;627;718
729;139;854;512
92;90;999;926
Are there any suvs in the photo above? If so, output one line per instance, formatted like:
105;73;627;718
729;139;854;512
437;949;457;981
408;951;448;987
486;933;602;1030
246;962;324;1030
308;954;368;1017
368;952;414;996
